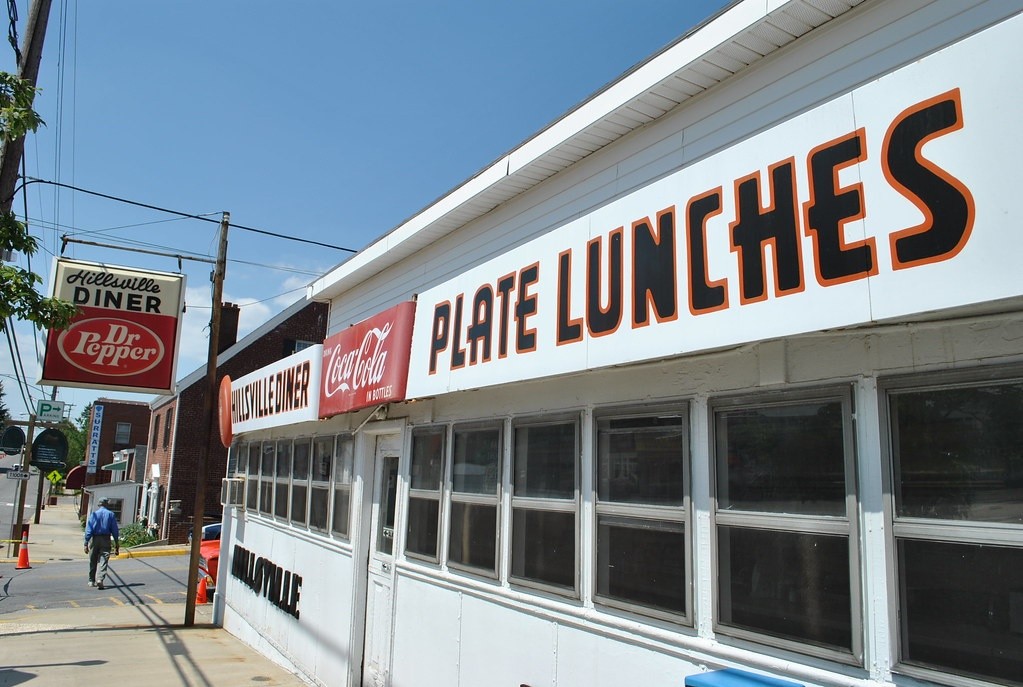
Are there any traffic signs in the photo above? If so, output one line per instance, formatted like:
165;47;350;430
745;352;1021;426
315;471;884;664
36;399;65;422
7;471;30;480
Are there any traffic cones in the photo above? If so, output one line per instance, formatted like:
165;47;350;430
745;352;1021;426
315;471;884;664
13;530;32;570
196;576;209;607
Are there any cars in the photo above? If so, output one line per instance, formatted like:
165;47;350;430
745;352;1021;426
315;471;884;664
187;522;222;546
194;539;220;603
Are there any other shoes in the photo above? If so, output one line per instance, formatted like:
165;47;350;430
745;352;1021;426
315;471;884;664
97;580;104;589
88;582;94;586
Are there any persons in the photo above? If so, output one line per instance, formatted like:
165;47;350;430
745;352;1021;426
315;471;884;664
83;496;119;590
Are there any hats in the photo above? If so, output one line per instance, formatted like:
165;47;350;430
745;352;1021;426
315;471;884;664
98;497;109;506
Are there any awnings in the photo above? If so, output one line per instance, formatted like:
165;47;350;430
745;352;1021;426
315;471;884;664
101;460;127;471
65;466;87;489
